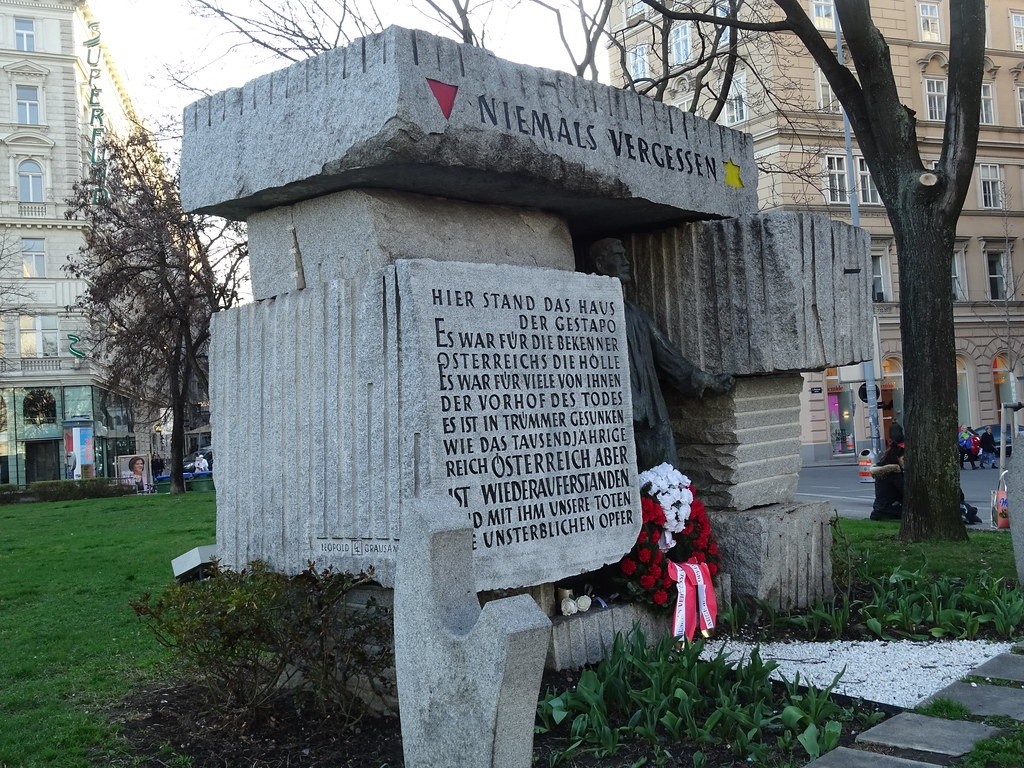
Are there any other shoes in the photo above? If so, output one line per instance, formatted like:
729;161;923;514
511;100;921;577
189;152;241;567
972;467;979;470
961;467;966;470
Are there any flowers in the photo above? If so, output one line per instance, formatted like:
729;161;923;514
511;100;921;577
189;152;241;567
603;460;723;613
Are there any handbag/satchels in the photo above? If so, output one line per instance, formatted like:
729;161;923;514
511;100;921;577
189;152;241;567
991;470;1010;529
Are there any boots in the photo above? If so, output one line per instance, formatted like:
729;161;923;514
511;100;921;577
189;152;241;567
980;463;985;469
992;463;998;468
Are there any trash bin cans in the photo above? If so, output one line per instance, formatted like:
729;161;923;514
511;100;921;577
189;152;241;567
857;449;879;483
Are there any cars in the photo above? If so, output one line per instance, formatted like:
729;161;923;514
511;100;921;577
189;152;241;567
958;424;1024;463
183;449;214;472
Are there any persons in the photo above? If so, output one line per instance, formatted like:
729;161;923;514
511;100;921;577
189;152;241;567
123;457;148;490
151;454;166;481
960;491;982;523
194;455;209;472
870;442;904;521
889;418;904;443
980;426;999;469
958;424;979;471
590;237;735;475
